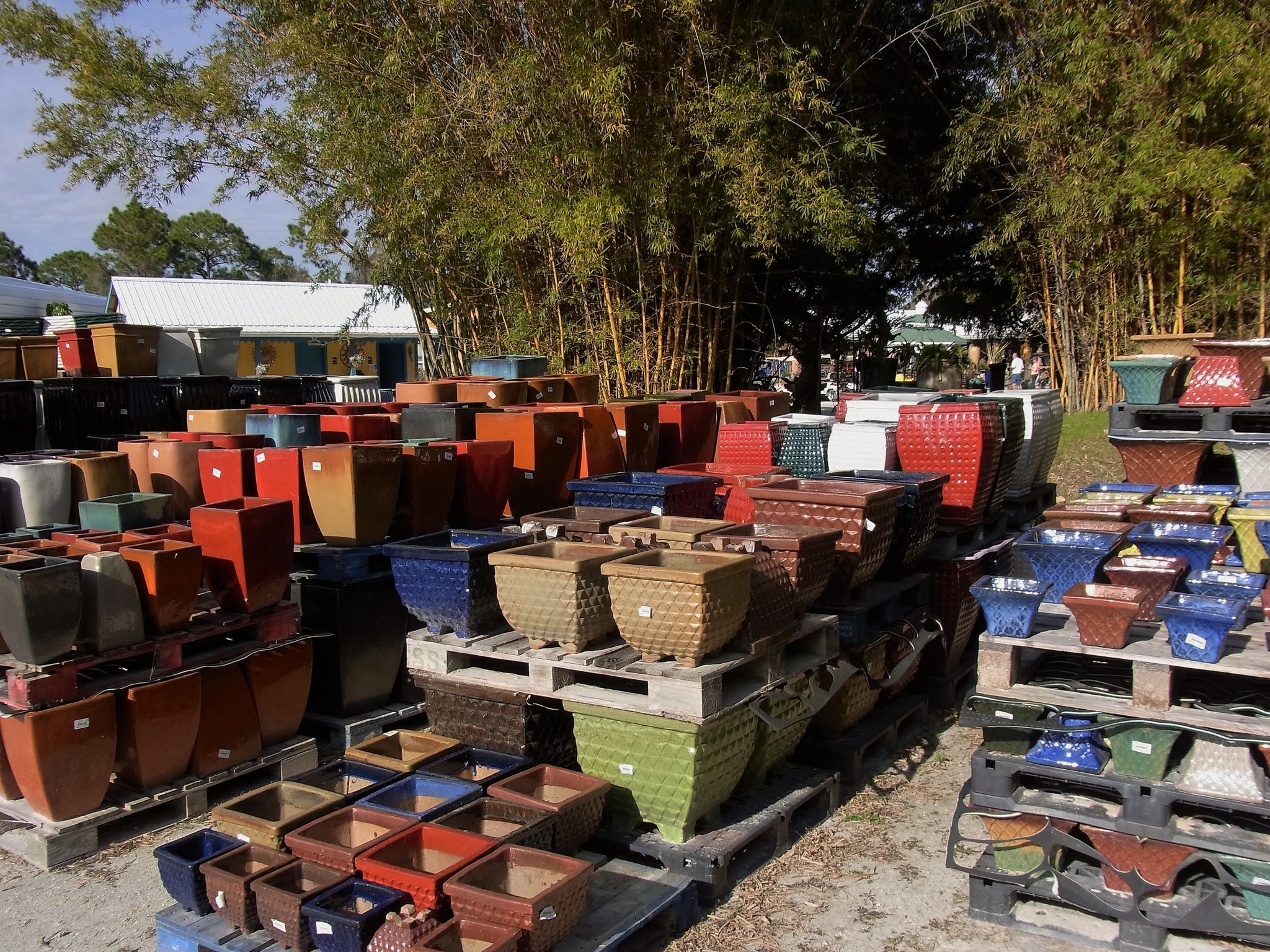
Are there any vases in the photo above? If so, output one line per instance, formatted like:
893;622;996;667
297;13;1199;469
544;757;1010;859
0;313;1270;952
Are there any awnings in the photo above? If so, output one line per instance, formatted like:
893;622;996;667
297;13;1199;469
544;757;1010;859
884;326;969;347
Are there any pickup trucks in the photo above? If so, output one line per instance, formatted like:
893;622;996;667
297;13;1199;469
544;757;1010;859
821;372;857;402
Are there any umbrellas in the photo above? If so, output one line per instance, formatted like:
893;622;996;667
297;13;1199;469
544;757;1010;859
899;314;949;324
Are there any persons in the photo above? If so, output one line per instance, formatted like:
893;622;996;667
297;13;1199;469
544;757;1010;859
833;345;922;390
1008;353;1024;390
1029;357;1039;389
